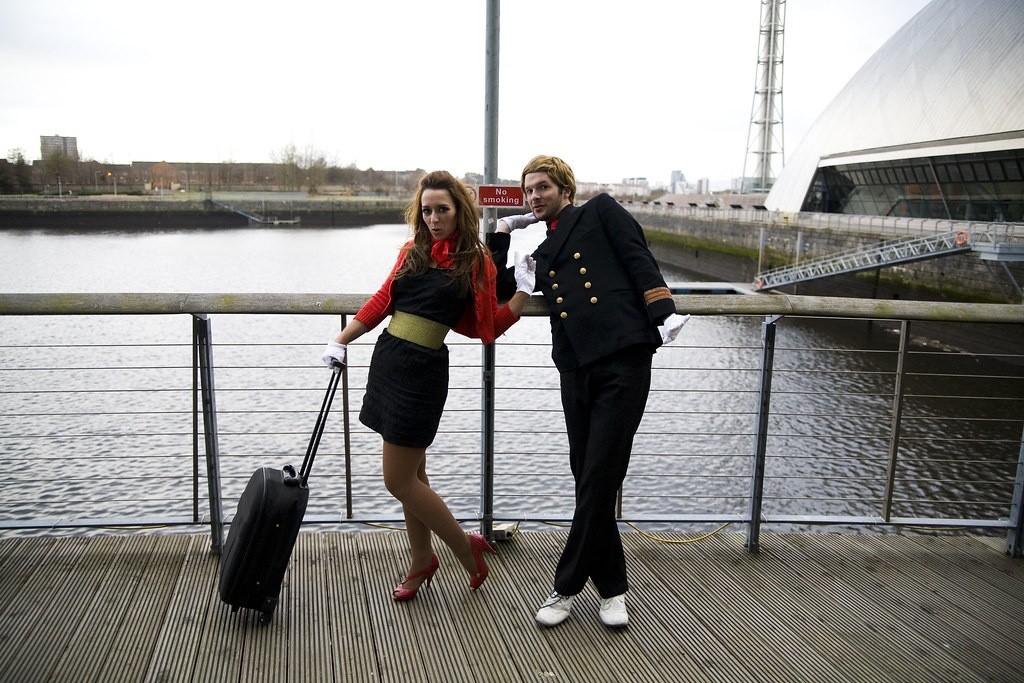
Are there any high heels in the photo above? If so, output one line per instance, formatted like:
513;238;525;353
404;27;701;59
469;534;497;591
392;554;440;601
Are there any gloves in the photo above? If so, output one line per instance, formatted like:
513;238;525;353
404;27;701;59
322;341;347;376
663;313;690;344
498;211;538;232
513;250;536;297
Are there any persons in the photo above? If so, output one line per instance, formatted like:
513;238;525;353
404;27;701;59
485;155;690;625
321;171;537;603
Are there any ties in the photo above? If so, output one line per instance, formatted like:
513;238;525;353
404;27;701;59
549;219;559;231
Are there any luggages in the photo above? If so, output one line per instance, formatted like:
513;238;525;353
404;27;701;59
218;358;346;626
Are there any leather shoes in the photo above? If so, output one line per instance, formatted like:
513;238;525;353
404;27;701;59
534;588;575;627
599;593;629;628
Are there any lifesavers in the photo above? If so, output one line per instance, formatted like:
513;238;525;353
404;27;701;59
956;230;967;245
